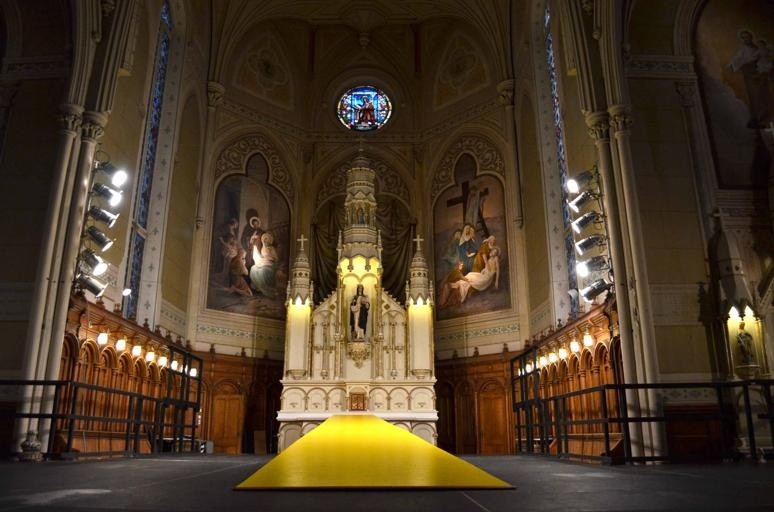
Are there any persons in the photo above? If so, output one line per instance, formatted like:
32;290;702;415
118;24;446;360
751;35;774;74
436;259;469;310
212;217;239;273
247;232;280;299
239;214;267;268
228;248;254;299
457;224;481;273
722;28;774;128
349;284;370;341
217;232;234;287
733;321;756;366
354;94;377;124
439;229;462;273
471;235;497;272
448;245;502;306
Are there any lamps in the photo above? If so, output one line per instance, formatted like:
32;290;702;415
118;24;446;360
566;166;611;303
97;331;198;380
517;325;593;376
78;152;127;302
